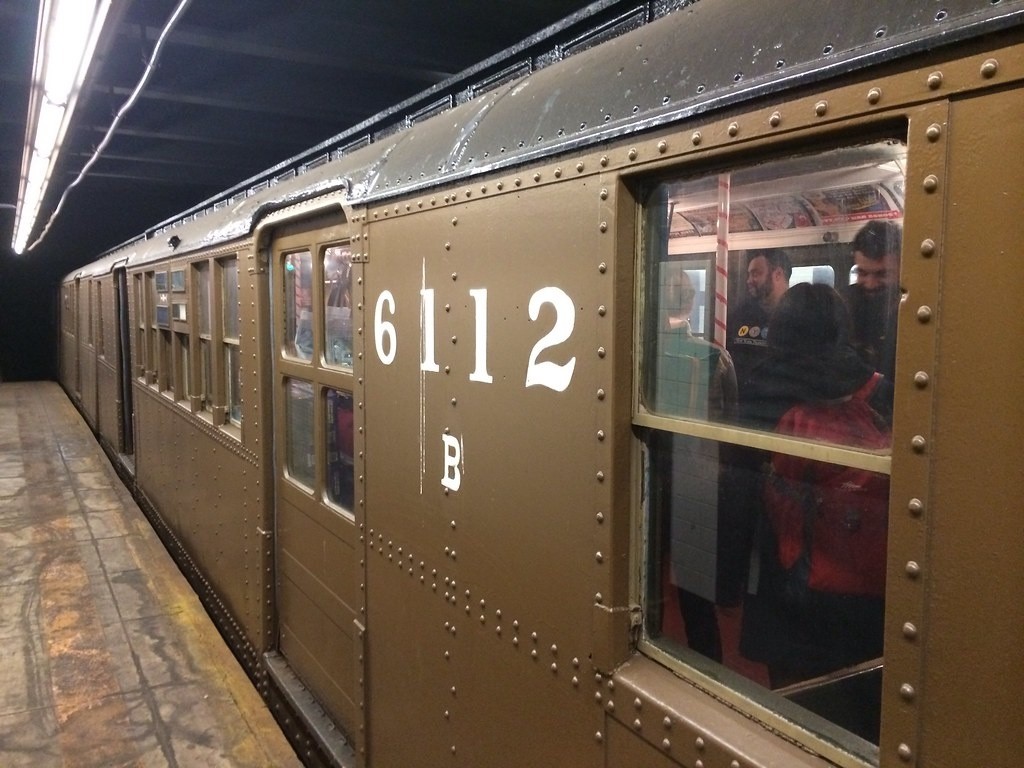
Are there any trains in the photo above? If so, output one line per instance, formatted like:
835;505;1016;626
59;0;1024;768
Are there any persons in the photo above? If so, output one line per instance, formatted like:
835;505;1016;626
842;220;901;413
727;248;793;396
645;271;748;666
741;282;894;746
335;395;354;491
